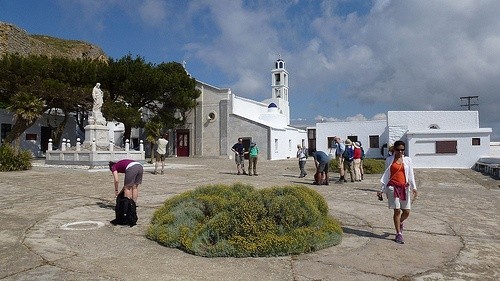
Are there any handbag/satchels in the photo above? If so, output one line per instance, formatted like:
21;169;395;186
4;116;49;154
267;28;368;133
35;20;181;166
342;149;350;159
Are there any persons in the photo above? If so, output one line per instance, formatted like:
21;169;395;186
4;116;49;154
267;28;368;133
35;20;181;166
244;141;259;176
377;140;418;244
109;159;143;205
296;136;365;185
231;137;248;174
154;134;168;175
92;83;104;112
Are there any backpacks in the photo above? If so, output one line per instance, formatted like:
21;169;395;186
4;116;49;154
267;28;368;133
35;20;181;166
304;149;309;159
114;198;137;226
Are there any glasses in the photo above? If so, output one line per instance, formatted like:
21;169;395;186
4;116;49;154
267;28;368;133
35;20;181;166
395;148;405;152
240;141;242;142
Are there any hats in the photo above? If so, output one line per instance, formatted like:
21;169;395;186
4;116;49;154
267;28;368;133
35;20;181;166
251;142;256;145
297;145;301;148
353;141;360;148
344;139;352;146
334;135;340;140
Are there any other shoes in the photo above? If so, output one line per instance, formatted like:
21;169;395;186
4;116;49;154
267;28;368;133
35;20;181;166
344;180;347;182
298;174;307;178
237;172;240;175
313;182;320;185
400;223;403;233
335;180;344;183
355;179;361;181
254;174;258;176
243;172;248;175
351;180;354;182
161;170;164;174
249;174;252;176
395;234;404;243
325;181;329;185
154;171;158;174
